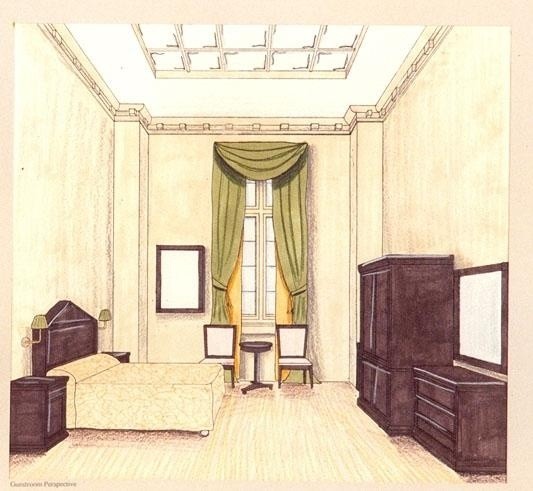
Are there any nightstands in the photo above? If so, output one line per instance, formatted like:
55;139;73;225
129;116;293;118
101;352;130;364
9;376;69;453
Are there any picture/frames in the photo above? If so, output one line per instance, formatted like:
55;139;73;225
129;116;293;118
454;263;506;373
154;243;205;315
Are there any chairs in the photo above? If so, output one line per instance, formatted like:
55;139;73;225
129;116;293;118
275;325;314;391
412;365;506;473
198;324;236;391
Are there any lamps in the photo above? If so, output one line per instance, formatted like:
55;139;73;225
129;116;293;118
97;309;111;331
21;315;48;349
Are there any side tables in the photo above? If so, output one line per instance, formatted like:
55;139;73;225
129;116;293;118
238;340;273;395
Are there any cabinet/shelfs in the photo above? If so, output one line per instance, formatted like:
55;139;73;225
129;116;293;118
355;255;455;439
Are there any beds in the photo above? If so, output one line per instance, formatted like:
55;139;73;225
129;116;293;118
31;300;225;434
